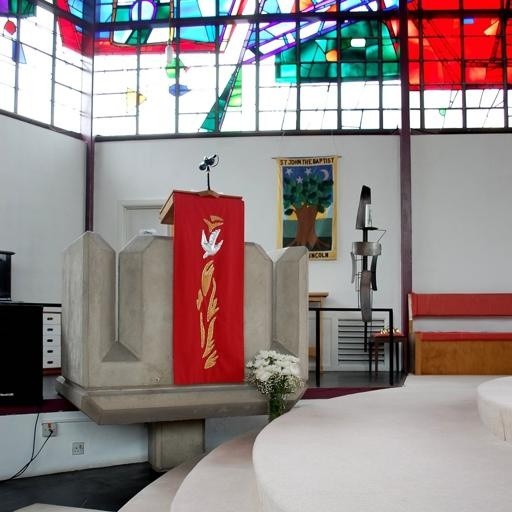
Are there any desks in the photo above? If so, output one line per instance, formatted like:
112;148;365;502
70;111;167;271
371;333;407;378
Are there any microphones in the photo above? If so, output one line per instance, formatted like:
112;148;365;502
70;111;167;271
199;155;216;170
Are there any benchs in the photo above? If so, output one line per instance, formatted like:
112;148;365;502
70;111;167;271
407;292;512;376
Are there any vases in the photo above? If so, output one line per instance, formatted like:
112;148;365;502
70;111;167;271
266;395;283;422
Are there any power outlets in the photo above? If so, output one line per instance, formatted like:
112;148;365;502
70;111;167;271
71;442;84;455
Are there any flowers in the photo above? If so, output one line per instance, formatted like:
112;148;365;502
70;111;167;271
244;351;305;415
